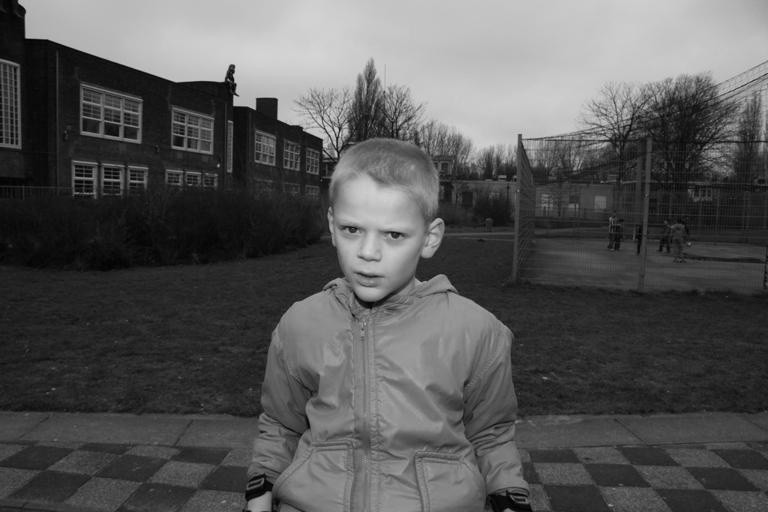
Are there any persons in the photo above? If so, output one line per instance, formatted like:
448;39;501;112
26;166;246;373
633;222;648;255
607;212;618;252
656;220;672;252
672;219;688;263
243;135;537;511
611;218;624;249
681;223;696;246
225;63;239;98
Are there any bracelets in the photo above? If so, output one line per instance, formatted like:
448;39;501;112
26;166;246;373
492;489;533;509
243;473;275;500
